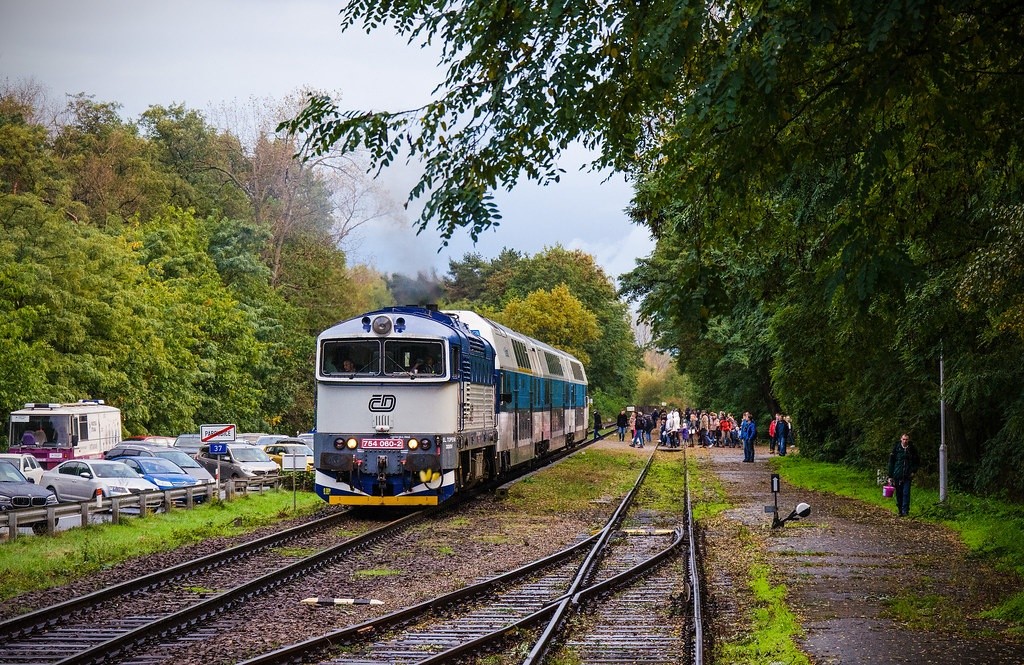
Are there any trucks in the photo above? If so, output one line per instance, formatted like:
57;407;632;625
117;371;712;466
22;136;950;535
9;396;122;473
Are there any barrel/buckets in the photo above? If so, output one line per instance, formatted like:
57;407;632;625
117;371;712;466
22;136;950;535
882;481;895;497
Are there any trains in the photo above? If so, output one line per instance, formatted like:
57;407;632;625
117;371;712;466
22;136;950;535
311;302;591;515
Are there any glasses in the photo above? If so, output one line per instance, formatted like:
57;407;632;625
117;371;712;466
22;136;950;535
593;411;595;413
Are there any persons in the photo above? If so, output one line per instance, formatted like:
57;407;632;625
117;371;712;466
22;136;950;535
20;425;36;445
342;358;357;373
410;354;438;374
629;405;756;450
886;433;921;517
743;414;756;463
768;412;795;455
593;409;604;443
617;409;627;441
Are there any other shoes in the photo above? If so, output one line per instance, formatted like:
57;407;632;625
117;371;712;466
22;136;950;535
901;513;908;517
708;444;742;449
779;452;785;456
698;442;702;445
670;444;677;448
743;460;753;463
688;443;694;447
658;440;662;442
638;445;643;448
769;449;774;454
629;444;635;447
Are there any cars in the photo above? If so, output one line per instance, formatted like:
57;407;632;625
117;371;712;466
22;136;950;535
1;432;314;530
